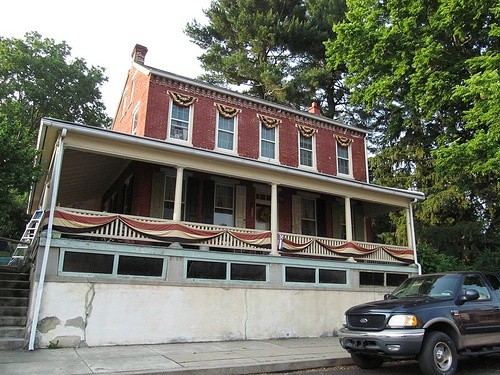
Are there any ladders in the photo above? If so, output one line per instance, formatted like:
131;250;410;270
6;209;45;267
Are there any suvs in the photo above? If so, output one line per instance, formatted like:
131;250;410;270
336;272;500;375
